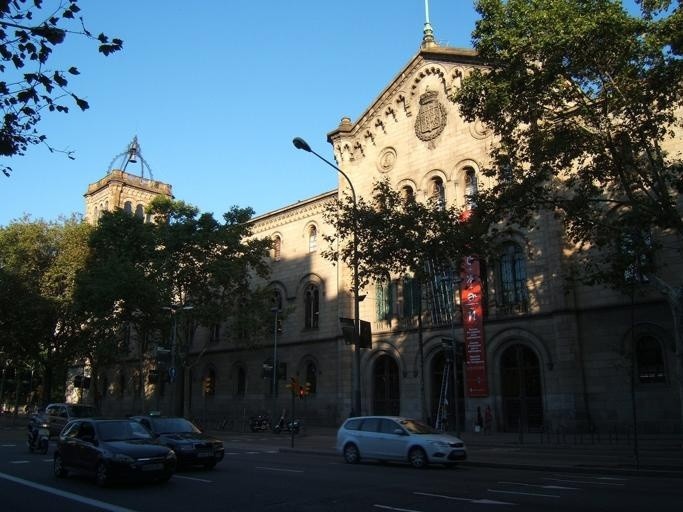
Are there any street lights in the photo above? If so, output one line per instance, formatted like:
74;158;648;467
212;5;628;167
292;137;361;417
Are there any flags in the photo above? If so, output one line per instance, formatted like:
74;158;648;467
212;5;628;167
337;317;360;346
358;319;372;349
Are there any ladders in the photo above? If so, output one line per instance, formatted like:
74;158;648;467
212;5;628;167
435;364;450;430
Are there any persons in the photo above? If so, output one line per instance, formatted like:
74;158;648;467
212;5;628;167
28;408;50;450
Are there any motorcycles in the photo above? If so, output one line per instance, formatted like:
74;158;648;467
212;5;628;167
249;414;306;435
24;416;52;456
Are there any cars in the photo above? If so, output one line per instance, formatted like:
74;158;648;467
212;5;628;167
45;402;226;487
334;415;470;470
